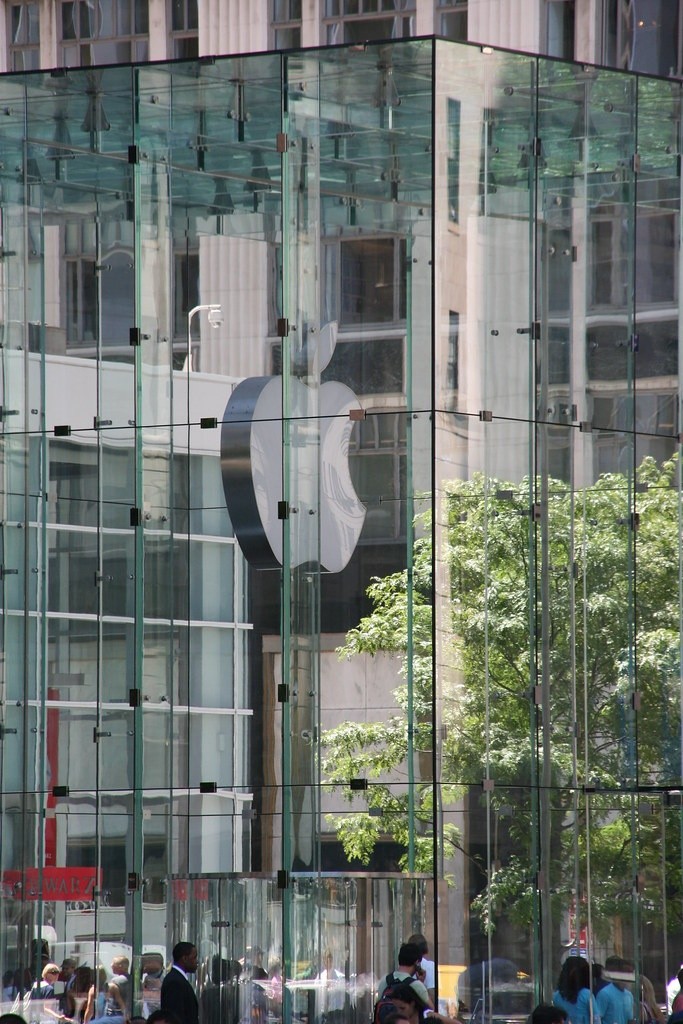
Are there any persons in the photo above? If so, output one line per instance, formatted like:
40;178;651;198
592;955;683;1024
528;956;600;1024
0;1014;26;1024
126;1010;170;1024
458;958;519;1015
2;939;164;1024
160;942;199;1024
373;934;462;1024
668;969;683;1024
199;940;379;1024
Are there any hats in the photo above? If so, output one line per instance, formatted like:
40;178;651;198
60;959;76;968
399;944;422;958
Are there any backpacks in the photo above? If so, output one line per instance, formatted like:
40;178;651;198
374;971;419;1024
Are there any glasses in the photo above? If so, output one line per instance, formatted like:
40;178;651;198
48;970;60;976
